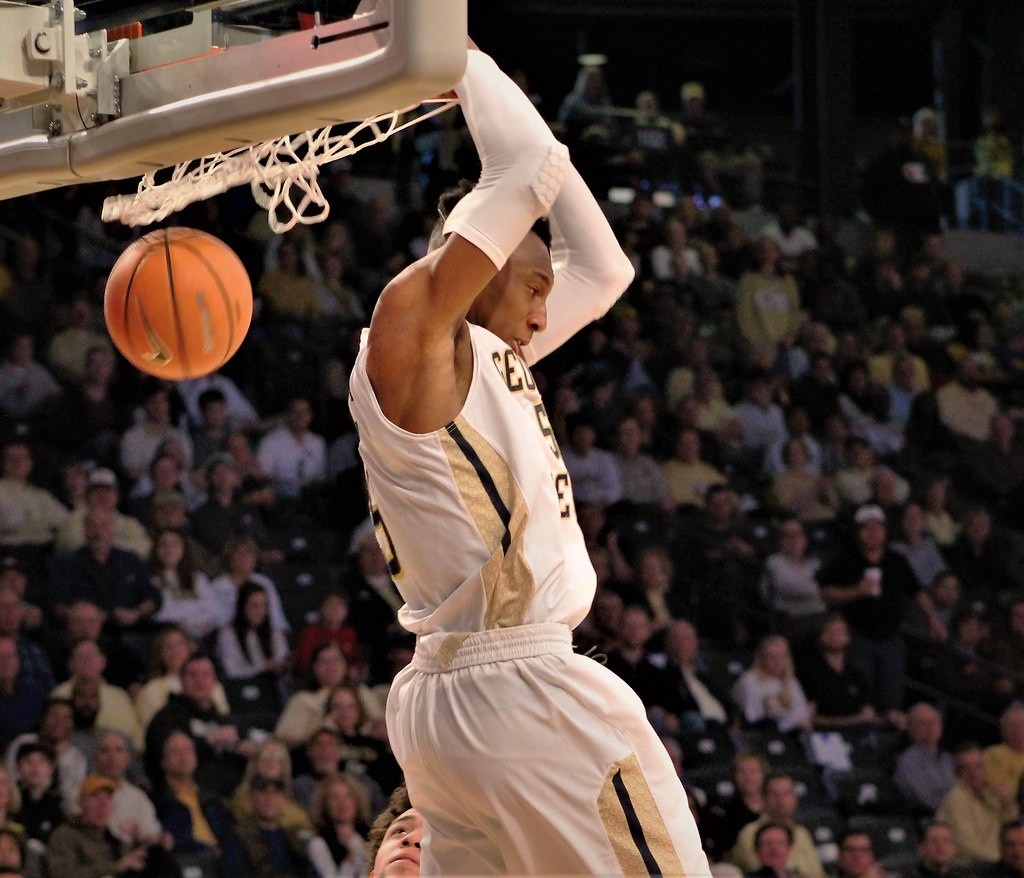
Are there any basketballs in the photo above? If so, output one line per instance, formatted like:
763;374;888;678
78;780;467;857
104;227;255;379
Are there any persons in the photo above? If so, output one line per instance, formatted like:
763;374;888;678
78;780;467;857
348;0;710;878
365;784;424;878
0;52;1024;878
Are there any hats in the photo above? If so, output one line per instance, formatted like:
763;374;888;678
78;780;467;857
82;468;119;488
854;503;886;527
13;740;45;764
77;774;117;799
242;776;285;802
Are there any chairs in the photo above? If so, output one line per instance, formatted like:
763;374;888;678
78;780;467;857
609;181;642;216
651;182;685;220
760;764;921;868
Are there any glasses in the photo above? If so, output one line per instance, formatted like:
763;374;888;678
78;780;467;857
843;844;873;857
